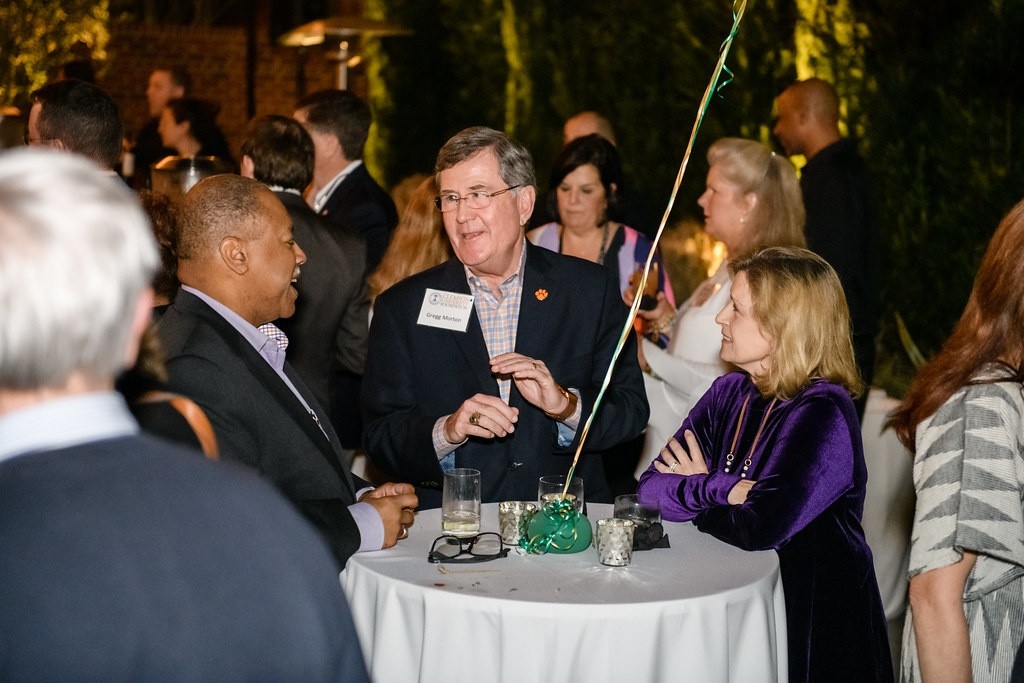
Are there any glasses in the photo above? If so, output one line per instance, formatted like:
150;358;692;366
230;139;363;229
433;183;538;212
22;132;60;148
427;532;510;565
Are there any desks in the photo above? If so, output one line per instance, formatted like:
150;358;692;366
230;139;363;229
338;501;787;683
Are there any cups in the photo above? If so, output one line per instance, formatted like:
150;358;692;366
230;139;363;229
614;491;662;531
596;517;634;567
538;474;584;518
442;467;481;546
499;501;538;547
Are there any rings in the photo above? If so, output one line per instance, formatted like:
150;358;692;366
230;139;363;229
533;363;536;369
398;528;408;539
469;410;481;425
670;461;678;471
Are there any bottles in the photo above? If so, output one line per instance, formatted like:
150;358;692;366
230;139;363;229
630;260;659;310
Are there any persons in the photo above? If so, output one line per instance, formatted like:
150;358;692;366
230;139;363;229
361;125;650;510
625;137;804;480
0;143;371;683
638;245;893;683
524;133;650;371
878;197;1024;683
773;78;890;425
564;111;617;146
27;54;454;482
155;175;419;576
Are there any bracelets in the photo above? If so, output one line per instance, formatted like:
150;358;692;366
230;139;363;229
545;385;577;421
